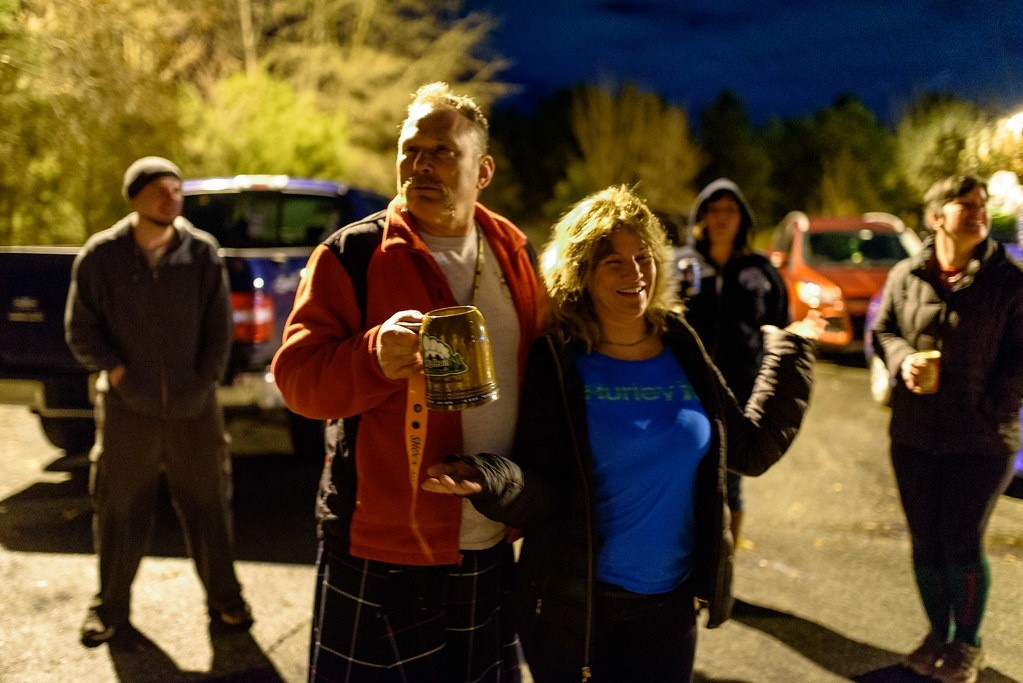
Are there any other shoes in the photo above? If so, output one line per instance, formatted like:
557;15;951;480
209;601;253;631
82;614;128;641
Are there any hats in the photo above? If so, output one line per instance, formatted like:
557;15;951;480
122;155;181;200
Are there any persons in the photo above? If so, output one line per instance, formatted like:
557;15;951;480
271;83;549;681
676;177;788;549
422;185;828;682
64;157;253;639
872;174;1023;683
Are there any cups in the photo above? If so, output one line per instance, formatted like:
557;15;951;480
913;349;941;394
394;305;500;412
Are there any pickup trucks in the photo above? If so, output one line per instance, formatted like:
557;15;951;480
0;175;395;469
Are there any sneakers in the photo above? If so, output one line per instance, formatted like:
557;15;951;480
931;636;983;683
903;632;945;675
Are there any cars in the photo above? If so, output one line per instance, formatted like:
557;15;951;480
654;210;702;294
866;241;1022;477
766;209;926;356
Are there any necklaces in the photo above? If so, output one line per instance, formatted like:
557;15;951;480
603;334;648;346
